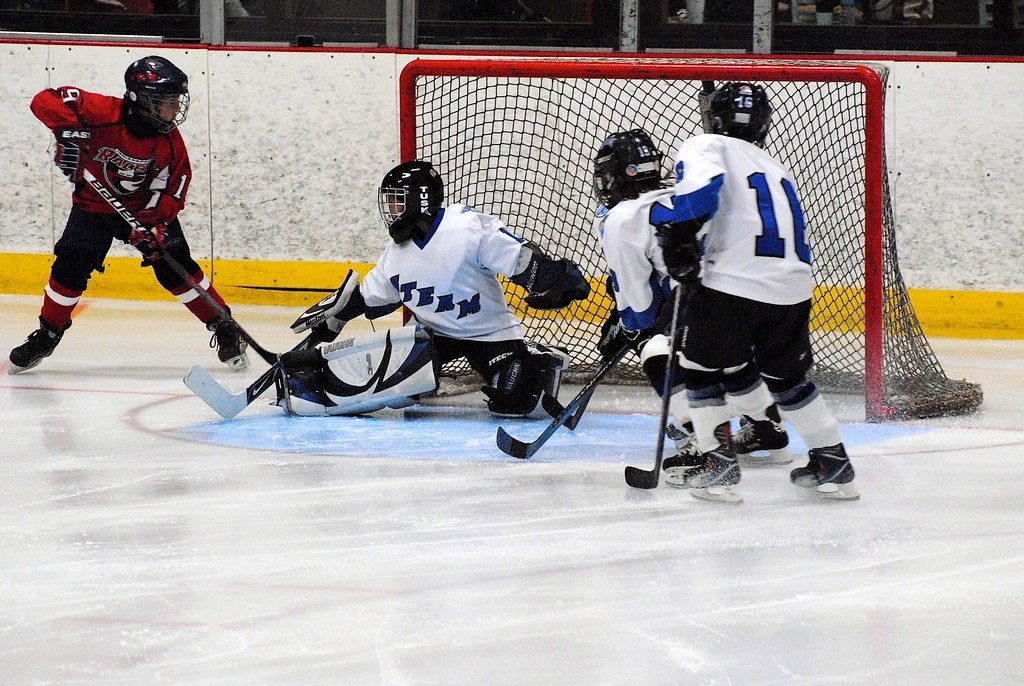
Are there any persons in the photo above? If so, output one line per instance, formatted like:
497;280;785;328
281;159;593;420
8;54;250;378
644;80;861;503
590;127;795;488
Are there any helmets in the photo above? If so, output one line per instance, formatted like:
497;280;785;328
595;131;662;208
123;57;188;137
378;162;444;241
708;86;771;142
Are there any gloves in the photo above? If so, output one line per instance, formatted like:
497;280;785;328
55;123;92;183
655;221;706;284
599;311;638;359
305;316;346;350
525;259;588;310
128;211;170;266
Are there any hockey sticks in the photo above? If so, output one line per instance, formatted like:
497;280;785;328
541;357;605;432
82;165;282;367
496;342;630;459
623;282;686;488
182;333;320;420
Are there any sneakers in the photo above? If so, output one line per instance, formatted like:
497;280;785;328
683;421;741;507
791;444;860;503
712;403;791;467
662;418;703;486
206;306;248;375
9;318;73;375
